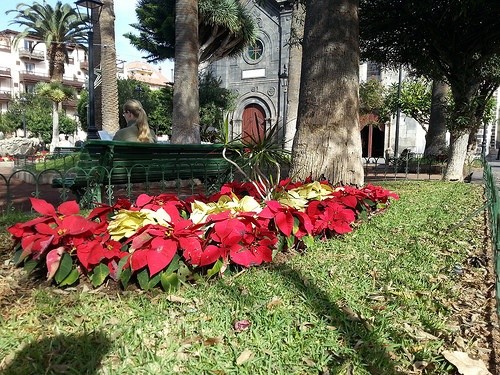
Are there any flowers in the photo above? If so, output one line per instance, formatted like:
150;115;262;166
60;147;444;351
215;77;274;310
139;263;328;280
10;177;399;293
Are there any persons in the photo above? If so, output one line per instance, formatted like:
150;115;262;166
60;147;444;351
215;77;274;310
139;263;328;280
60;135;73;148
113;99;159;145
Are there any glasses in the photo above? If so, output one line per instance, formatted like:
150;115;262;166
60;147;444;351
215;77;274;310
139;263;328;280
122;112;128;116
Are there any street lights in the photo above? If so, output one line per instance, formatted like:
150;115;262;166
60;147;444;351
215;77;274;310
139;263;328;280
20;97;30;138
73;0;102;148
280;64;289;152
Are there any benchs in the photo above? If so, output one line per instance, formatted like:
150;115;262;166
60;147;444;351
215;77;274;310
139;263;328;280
44;146;82;162
53;143;252;201
